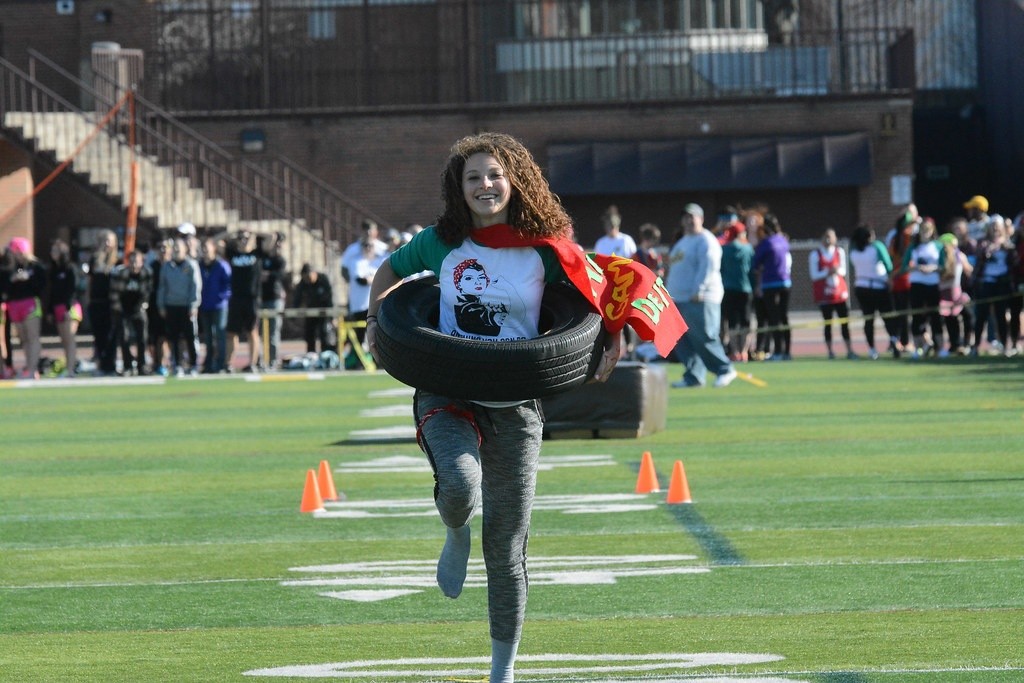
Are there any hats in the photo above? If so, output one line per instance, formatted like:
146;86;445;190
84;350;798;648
10;238;28;255
680;203;703;219
727;222;746;241
963;195;988;212
179;223;197;237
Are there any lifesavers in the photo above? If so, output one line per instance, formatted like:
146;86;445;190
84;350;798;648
372;273;609;403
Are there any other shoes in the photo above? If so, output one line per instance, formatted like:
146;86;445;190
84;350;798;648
0;358;282;380
731;348;792;362
713;367;738;387
827;338;1024;361
673;378;693;387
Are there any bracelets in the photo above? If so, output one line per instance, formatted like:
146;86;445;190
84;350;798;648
366;316;377;327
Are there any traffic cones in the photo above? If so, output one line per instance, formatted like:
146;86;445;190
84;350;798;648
317;460;338;500
635;452;659;493
667;460;691;503
300;469;324;512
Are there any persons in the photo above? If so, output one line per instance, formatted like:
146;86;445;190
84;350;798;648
951;196;1024;357
0;237;49;378
712;203;792;362
630;223;664;281
110;240;153;377
89;228;121;376
225;230;286;373
593;206;641;360
849;221;903;360
147;234;233;376
367;131;622;683
664;203;738;389
293;264;333;352
341;218;424;369
884;201;975;359
808;227;861;360
50;239;83;377
